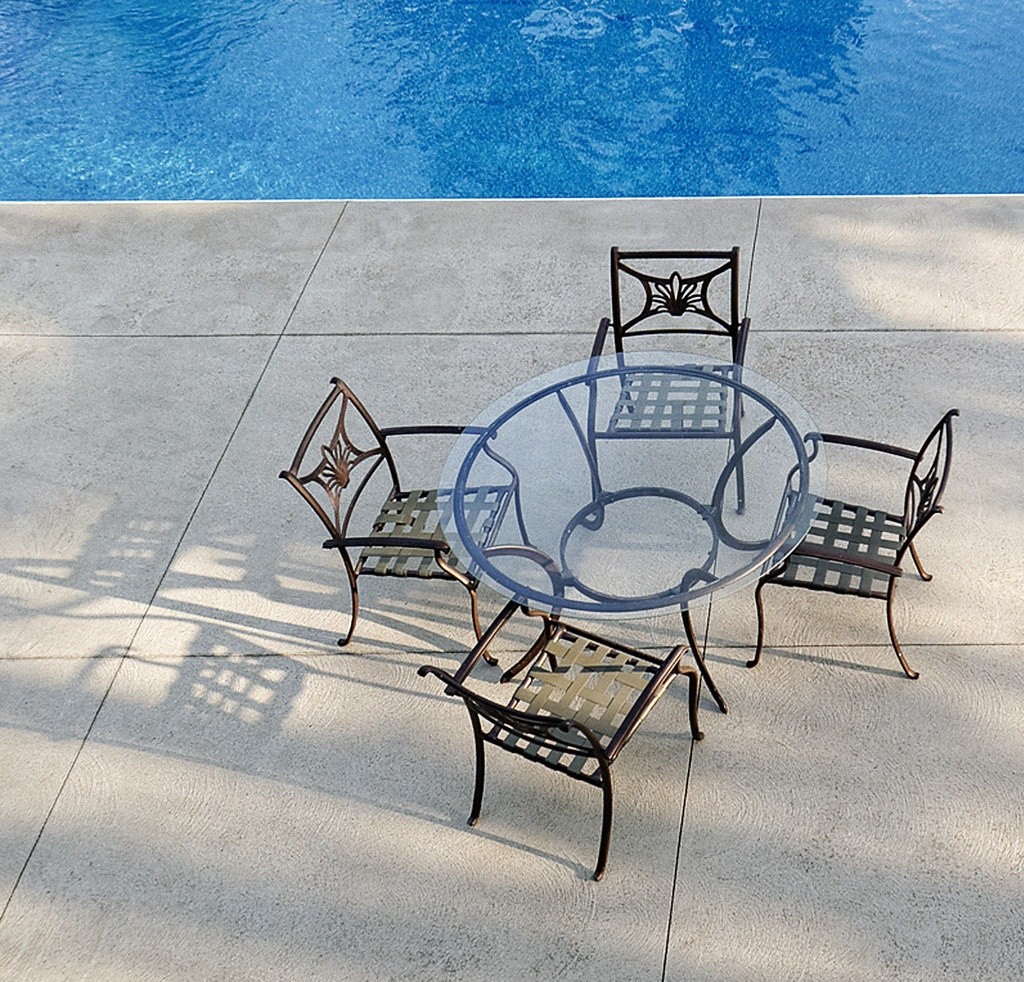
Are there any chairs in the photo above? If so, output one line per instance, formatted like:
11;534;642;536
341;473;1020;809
745;408;960;679
278;376;538;667
585;246;751;515
417;585;705;881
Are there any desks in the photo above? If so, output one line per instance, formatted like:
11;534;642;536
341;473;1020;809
436;350;828;714
